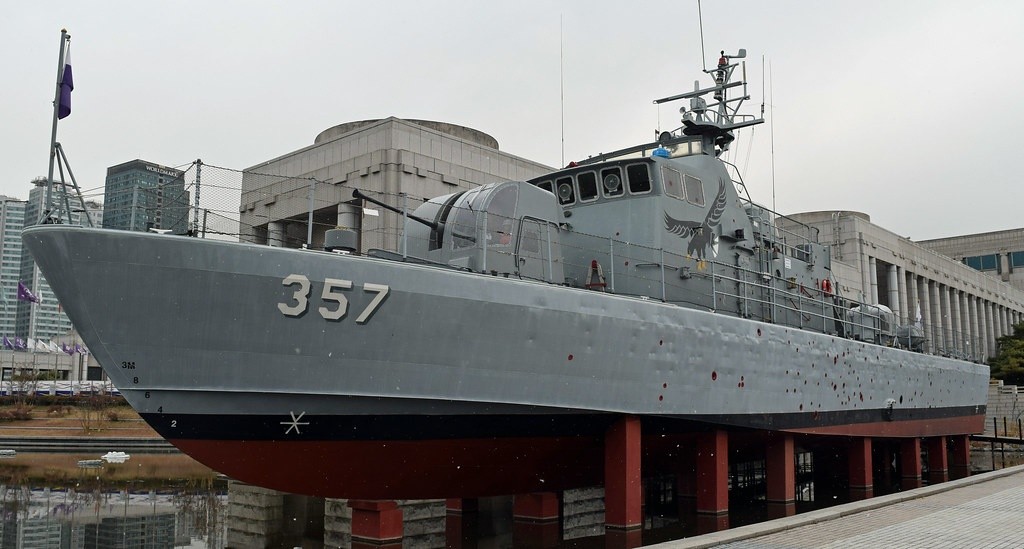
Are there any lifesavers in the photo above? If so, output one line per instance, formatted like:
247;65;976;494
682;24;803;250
822;279;832;296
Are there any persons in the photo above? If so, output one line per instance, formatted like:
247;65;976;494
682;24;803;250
499;220;513;243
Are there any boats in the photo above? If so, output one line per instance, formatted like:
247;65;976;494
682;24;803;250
18;44;990;499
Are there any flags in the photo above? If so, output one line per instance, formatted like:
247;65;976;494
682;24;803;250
52;42;74;119
3;335;87;356
17;282;38;304
